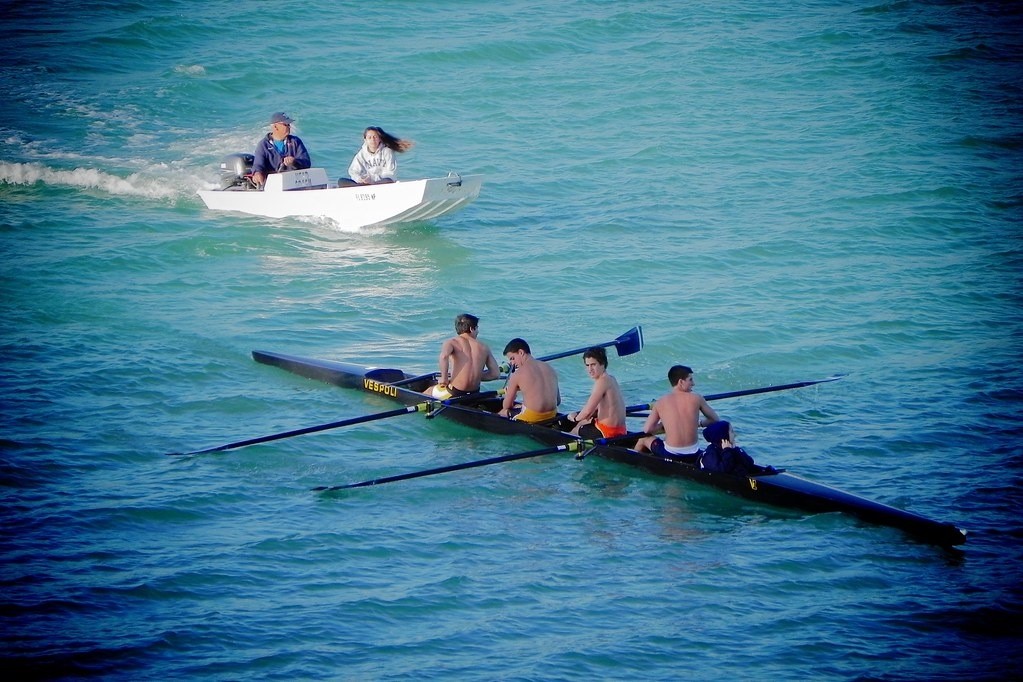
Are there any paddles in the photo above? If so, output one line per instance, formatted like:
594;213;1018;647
311;428;666;494
483;324;644;376
556;372;851;422
163;388;506;458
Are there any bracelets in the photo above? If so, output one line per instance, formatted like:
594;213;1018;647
700;420;702;427
574;416;577;422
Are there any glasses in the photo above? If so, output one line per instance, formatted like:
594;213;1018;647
278;122;290;126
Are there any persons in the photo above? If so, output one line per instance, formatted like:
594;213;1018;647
421;313;500;407
496;338;561;428
697;420;754;479
338;125;415;188
251;112;312;185
634;366;719;464
556;346;628;441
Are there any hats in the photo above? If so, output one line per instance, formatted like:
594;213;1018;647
272;112;295;124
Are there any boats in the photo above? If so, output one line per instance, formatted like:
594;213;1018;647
249;347;973;548
190;168;485;238
